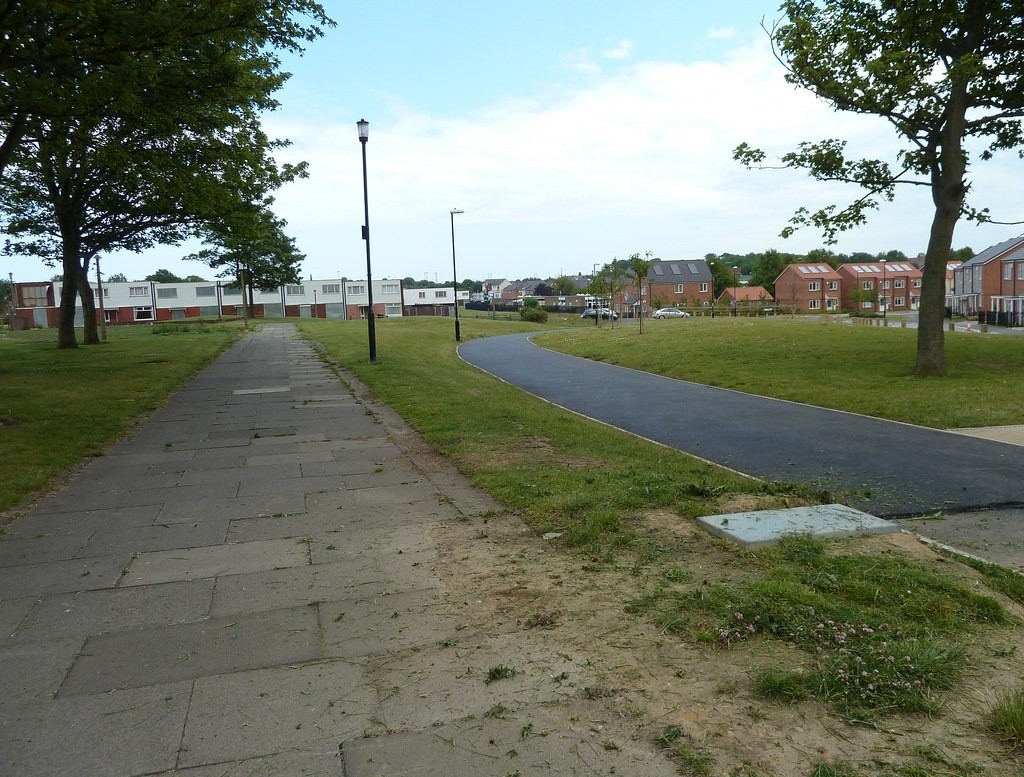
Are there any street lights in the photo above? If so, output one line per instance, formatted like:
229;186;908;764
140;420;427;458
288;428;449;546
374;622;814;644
450;208;464;341
93;253;109;343
733;266;738;318
880;259;887;318
357;119;376;365
314;290;317;319
710;262;714;318
593;263;600;275
9;272;16;316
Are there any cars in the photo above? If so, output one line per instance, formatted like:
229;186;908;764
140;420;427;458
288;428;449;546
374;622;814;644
652;308;690;319
581;308;618;322
756;306;774;316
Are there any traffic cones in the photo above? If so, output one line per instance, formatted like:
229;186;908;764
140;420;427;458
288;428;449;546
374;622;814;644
965;320;972;331
